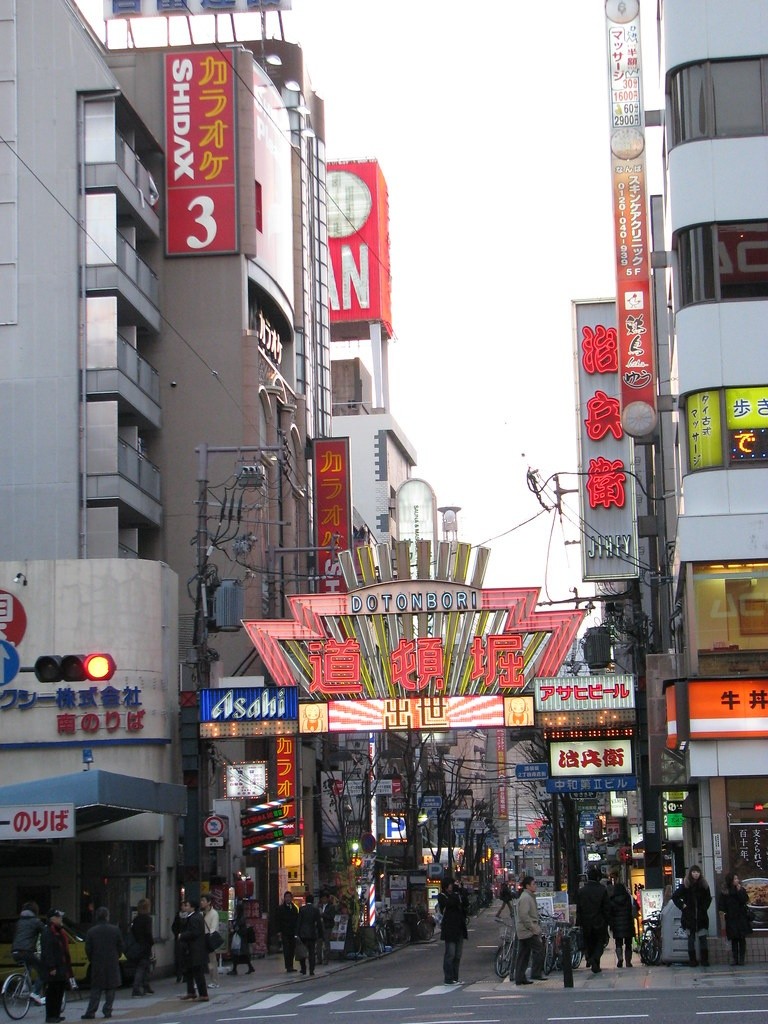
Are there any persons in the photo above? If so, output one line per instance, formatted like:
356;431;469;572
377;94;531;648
171;893;220;1001
39;909;75;1023
576;868;640;973
496;883;514;918
718;871;753;966
277;891;298;972
11;910;45;1003
672;865;712;967
437;877;468;986
227;905;255;975
515;876;548;985
81;907;124;1019
295;894;323;975
315;893;335;965
129;898;155;996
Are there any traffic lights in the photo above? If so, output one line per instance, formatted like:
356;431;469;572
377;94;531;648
33;651;119;684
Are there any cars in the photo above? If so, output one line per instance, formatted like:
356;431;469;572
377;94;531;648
494;880;522;900
0;907;136;989
578;873;588;889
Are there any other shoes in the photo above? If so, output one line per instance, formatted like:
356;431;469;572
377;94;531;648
516;976;548;986
286;966;297;974
316;961;328;965
207;982;219;988
226;968;256;975
445;980;466;986
299;969;316;976
176;976;186;983
180;993;209;1002
46;1016;66;1023
81;1012;96;1019
105;1013;112;1018
132;984;155;998
586;960;602;973
30;992;41;1004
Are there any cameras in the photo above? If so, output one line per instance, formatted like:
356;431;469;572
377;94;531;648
451;882;464;892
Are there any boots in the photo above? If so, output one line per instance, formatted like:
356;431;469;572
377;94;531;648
686;949;710;967
615;948;633;967
730;951;745;965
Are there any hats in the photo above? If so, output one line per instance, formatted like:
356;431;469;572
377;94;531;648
47;908;65;918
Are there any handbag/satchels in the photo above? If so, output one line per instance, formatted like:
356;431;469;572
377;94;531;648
247;927;255;942
747;908;755;920
295;943;309;960
231;933;242;949
631;906;640;919
206;931;224;953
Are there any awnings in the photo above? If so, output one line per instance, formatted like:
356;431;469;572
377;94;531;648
377;844;418;870
0;770;187;841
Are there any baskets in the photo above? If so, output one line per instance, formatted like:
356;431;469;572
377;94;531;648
498;927;513;940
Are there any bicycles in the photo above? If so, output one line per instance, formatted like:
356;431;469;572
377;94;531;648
494;903;583;979
639;909;664;967
464;916;471;926
1;952;67;1021
315;903;436;960
